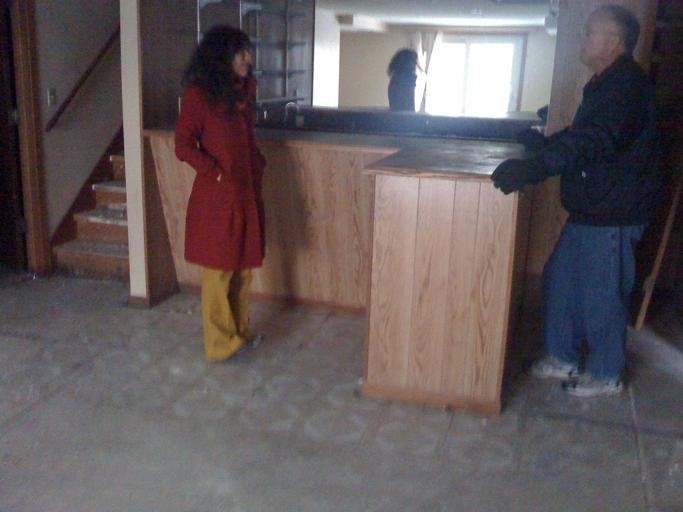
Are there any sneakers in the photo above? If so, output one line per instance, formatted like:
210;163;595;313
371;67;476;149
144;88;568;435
527;358;623;396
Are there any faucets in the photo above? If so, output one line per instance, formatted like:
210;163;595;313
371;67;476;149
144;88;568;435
282;99;299;127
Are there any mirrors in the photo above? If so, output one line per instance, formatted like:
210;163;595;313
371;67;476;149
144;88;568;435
196;0;558;123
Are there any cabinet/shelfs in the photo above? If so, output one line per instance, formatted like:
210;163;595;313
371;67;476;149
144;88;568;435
238;1;314;109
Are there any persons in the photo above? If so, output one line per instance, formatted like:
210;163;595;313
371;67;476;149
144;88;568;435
491;4;665;396
173;23;268;362
387;48;418;112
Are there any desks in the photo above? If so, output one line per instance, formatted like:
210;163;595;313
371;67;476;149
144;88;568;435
143;122;530;419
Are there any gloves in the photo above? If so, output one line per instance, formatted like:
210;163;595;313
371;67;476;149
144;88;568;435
490;159;544;193
518;129;544;146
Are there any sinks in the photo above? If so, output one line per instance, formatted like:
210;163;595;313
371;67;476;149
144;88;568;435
254;124;298;141
